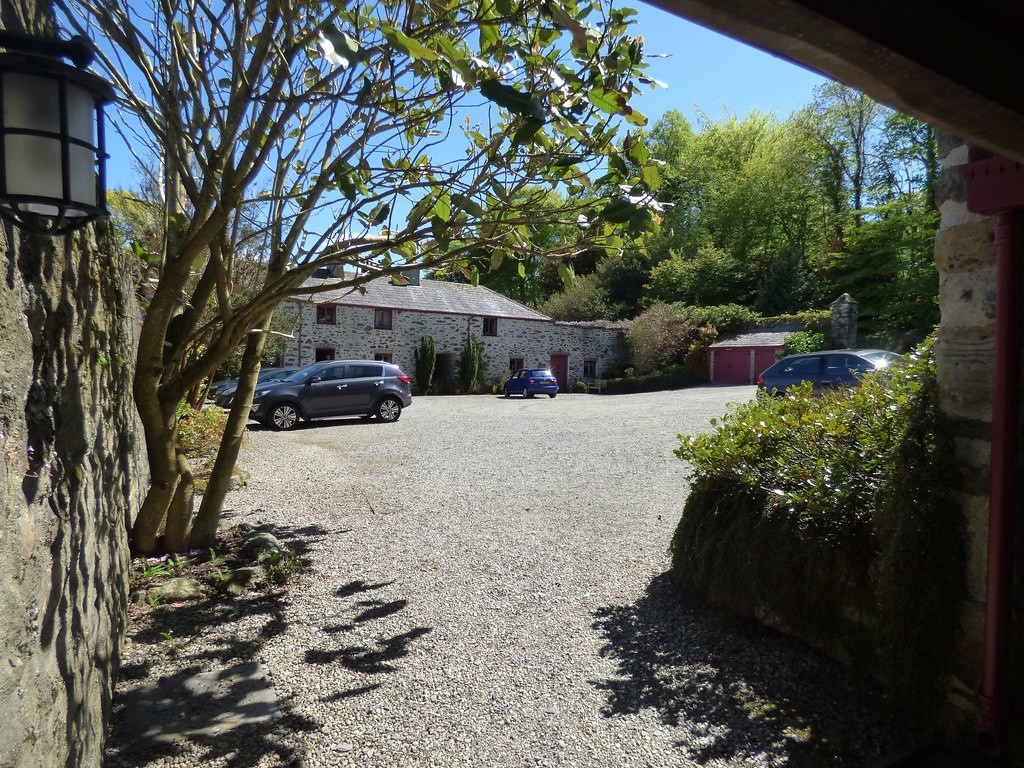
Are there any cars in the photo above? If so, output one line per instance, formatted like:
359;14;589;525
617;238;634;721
208;368;278;395
214;367;304;409
757;348;909;400
503;367;557;399
250;359;412;429
